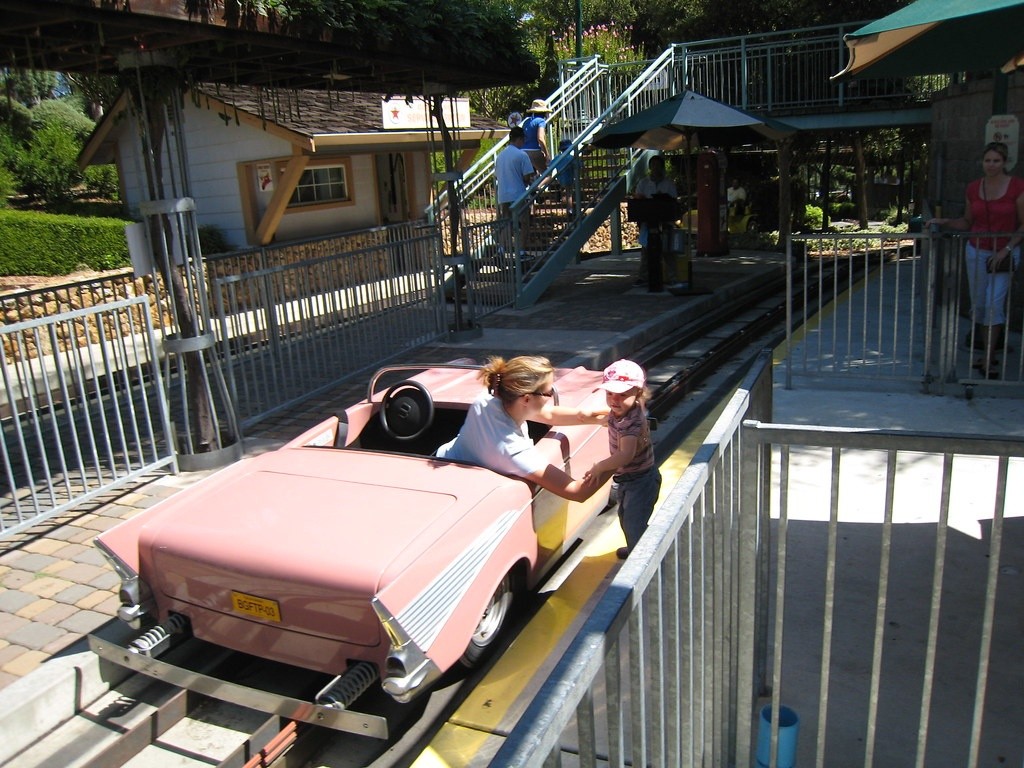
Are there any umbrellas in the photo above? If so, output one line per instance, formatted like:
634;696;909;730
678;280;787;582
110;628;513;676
828;0;1024;172
583;90;797;289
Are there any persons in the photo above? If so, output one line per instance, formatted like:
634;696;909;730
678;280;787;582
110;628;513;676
494;127;541;271
546;139;581;216
576;359;662;559
518;99;553;216
727;178;746;203
632;155;681;289
923;142;1024;380
436;357;649;504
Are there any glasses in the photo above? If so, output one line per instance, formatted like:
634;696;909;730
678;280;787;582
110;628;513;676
518;388;554;397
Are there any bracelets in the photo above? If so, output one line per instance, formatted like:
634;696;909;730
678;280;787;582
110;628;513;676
1006;245;1011;251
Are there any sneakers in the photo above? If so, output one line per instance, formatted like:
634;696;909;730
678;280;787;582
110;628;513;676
632;279;648;287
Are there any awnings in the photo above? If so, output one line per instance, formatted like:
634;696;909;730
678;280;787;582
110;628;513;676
770;108;932;130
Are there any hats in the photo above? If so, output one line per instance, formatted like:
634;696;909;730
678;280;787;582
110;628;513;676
526;99;553;112
591;359;645;393
559;140;573;150
509;127;527;137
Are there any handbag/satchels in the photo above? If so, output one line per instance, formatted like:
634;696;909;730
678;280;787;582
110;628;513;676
985;255;1015;273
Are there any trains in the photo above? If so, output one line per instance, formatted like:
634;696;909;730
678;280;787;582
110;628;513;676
92;358;653;704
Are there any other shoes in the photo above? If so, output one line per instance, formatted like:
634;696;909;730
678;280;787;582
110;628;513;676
616;547;629;559
979;363;999;379
520;252;534;261
494;253;506;270
985;355;999;366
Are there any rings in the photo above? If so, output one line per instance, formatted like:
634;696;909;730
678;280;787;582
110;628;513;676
644;438;648;442
